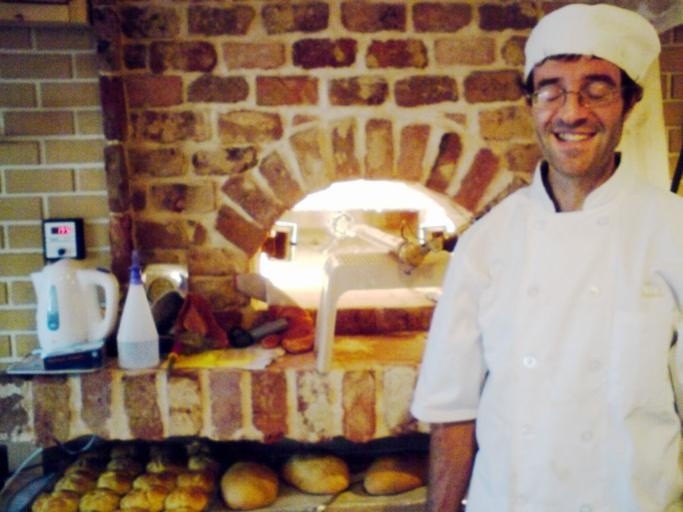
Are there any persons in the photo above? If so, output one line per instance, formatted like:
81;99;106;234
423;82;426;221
409;2;683;512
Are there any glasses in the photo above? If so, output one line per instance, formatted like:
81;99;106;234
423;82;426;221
527;86;623;109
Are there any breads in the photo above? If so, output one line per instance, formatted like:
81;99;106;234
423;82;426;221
32;441;427;512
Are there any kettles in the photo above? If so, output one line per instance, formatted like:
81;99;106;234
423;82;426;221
29;257;119;362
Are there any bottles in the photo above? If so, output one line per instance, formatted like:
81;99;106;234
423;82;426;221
117;250;161;371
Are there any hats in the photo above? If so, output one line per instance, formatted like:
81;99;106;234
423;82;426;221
521;1;664;88
